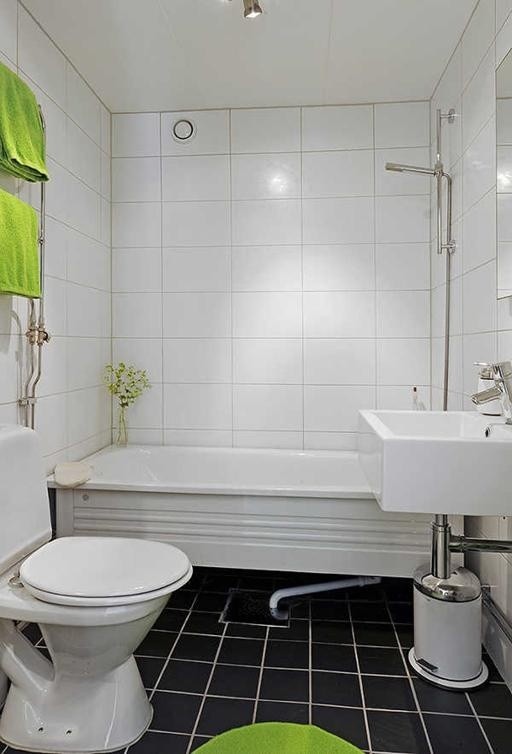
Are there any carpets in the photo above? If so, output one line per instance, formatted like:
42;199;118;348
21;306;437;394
191;719;364;754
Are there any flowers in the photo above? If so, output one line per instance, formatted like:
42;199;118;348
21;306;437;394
102;363;152;442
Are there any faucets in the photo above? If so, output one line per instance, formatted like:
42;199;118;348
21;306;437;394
468;361;511;427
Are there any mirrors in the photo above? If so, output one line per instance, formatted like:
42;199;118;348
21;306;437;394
496;50;511;300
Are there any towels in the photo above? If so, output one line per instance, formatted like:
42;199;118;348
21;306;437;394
1;64;50;299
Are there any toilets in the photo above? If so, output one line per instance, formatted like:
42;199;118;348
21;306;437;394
0;426;192;754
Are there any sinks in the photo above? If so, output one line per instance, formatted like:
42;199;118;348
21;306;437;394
355;408;511;517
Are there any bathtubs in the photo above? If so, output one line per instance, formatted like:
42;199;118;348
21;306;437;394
47;441;465;580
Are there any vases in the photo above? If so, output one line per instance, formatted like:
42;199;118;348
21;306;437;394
113;407;129;447
